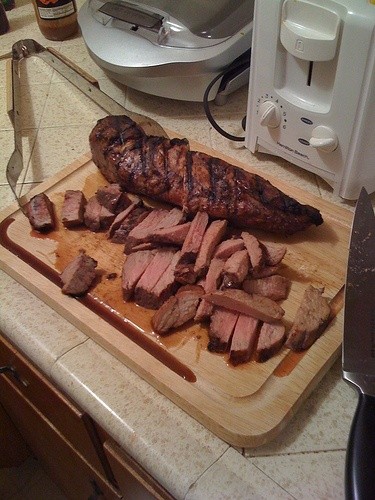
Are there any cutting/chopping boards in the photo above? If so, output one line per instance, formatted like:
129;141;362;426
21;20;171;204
0;127;355;449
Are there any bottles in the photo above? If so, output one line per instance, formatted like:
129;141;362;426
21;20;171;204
32;1;81;40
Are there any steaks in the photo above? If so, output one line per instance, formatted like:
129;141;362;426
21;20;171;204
25;115;333;365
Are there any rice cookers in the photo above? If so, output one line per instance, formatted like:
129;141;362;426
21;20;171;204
76;1;254;105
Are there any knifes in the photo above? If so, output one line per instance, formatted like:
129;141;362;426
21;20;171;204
341;185;375;500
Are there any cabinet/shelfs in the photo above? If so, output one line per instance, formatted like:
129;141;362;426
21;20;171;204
103;440;176;499
0;336;122;500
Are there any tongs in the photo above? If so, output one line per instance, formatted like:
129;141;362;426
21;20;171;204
5;39;170;217
